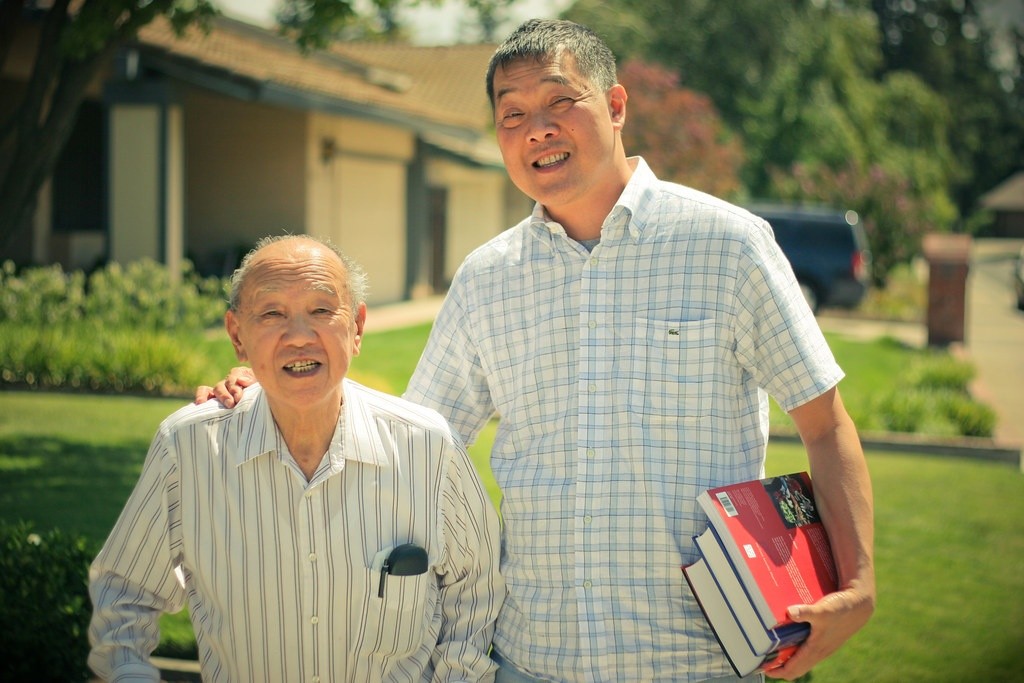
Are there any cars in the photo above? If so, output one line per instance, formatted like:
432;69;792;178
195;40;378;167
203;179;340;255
749;209;869;316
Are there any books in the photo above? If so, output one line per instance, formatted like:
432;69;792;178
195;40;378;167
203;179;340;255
679;471;841;679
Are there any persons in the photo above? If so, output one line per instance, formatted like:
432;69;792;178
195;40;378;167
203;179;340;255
194;20;877;682
88;233;503;682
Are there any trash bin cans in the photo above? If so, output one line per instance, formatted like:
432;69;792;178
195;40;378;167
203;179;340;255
924;238;968;346
